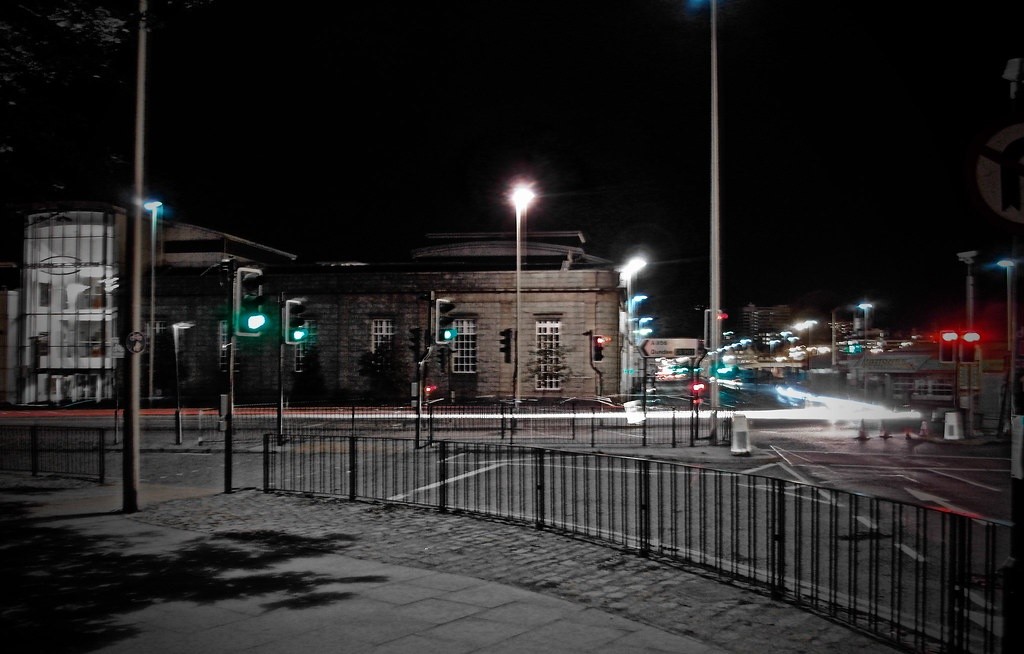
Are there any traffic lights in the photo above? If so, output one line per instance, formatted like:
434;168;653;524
284;299;310;345
940;329;957;364
592;333;605;363
498;329;512;363
232;267;268;336
434;298;457;345
960;330;978;364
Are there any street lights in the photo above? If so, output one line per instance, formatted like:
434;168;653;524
141;198;164;401
503;178;543;408
